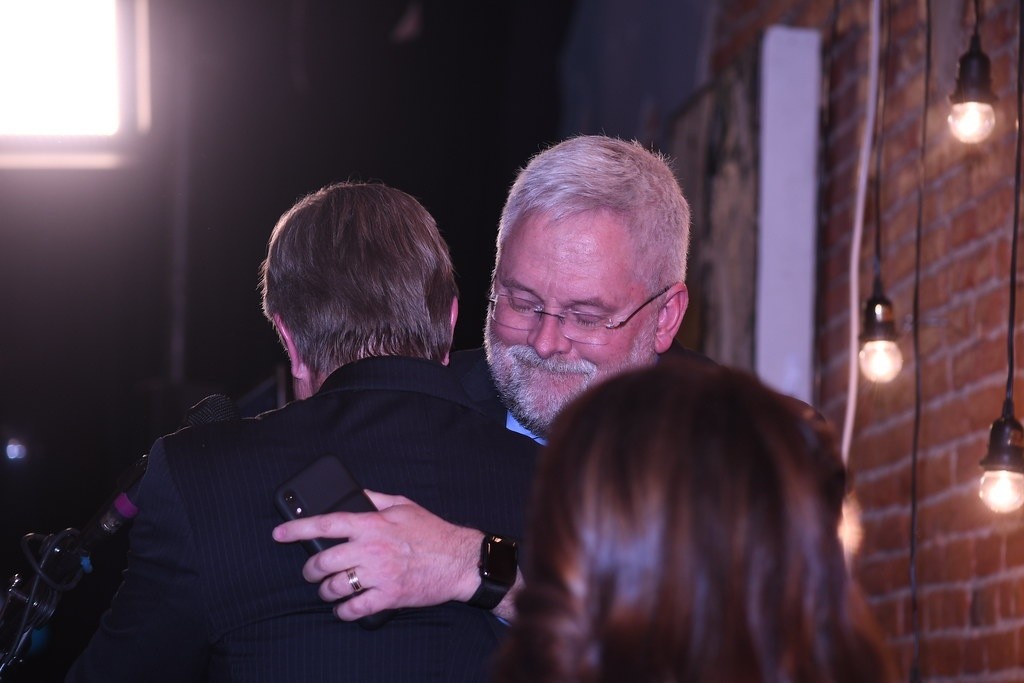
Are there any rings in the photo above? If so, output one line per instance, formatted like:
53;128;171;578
346;567;363;595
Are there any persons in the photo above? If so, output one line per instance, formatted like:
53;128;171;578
66;188;564;682
516;358;901;682
442;134;688;451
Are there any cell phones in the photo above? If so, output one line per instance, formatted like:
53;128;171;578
274;454;395;630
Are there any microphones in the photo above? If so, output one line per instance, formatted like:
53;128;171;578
73;394;240;561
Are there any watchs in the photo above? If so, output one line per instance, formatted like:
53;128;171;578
467;534;519;610
